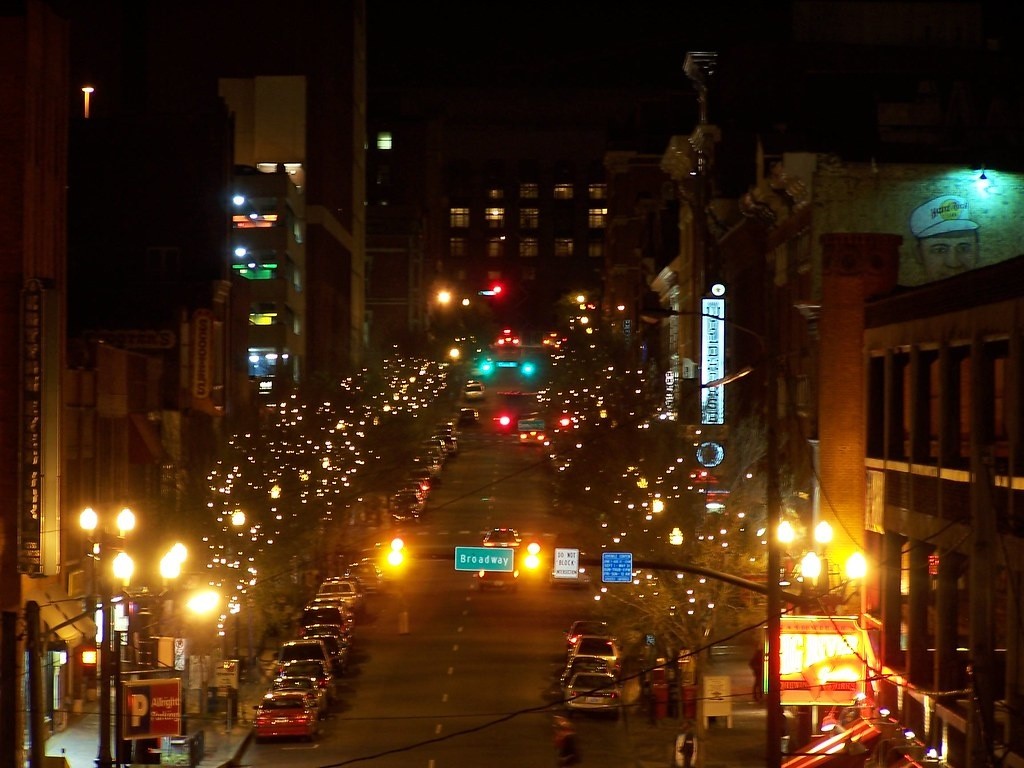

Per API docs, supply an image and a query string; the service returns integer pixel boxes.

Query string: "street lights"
[775,519,870,742]
[112,542,188,768]
[79,505,136,766]
[24,591,219,768]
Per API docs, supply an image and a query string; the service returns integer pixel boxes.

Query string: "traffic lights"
[560,417,569,425]
[500,415,510,426]
[384,536,406,568]
[524,539,543,571]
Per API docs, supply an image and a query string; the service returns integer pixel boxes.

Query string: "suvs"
[562,619,612,657]
[560,654,617,695]
[553,532,591,560]
[274,640,332,674]
[564,670,622,717]
[570,634,621,674]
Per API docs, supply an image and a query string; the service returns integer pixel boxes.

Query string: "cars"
[550,564,591,589]
[478,568,519,589]
[268,678,327,717]
[291,378,486,669]
[299,633,346,672]
[519,430,545,444]
[278,659,335,699]
[481,528,523,556]
[252,691,318,738]
